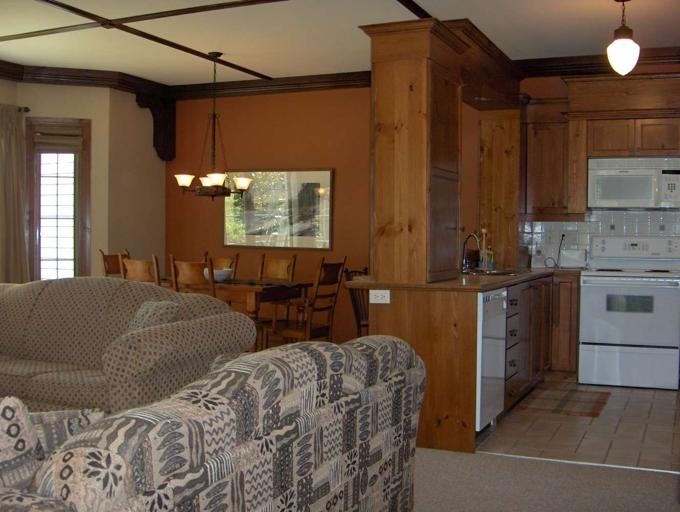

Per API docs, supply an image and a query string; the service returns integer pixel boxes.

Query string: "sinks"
[467,270,522,276]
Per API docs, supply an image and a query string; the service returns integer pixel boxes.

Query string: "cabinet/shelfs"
[587,73,680,157]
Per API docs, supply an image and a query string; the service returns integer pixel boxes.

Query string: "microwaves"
[586,156,680,209]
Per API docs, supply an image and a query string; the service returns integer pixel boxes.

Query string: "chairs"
[99,248,369,353]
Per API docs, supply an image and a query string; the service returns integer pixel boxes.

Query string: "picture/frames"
[222,168,335,251]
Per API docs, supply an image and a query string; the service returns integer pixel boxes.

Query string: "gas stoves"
[582,259,680,276]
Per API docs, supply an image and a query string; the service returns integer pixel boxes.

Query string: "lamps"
[607,0,640,76]
[174,51,253,201]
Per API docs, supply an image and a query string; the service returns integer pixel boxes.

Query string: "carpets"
[518,387,611,418]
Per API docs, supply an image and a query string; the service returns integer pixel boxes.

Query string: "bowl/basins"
[203,268,233,282]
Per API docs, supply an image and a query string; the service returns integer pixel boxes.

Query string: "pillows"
[0,395,105,492]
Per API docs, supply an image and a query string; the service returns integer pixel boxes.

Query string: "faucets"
[464,232,482,275]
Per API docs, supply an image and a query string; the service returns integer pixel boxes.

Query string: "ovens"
[579,275,679,348]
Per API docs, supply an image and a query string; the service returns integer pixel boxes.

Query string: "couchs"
[0,276,257,417]
[0,334,425,512]
